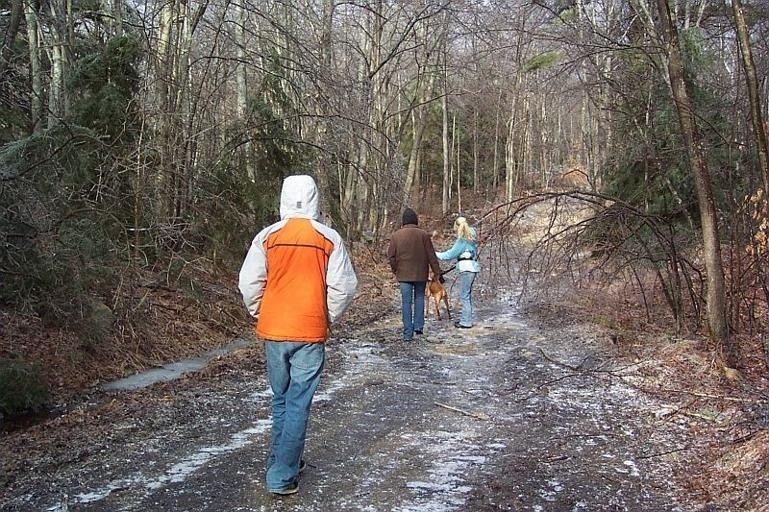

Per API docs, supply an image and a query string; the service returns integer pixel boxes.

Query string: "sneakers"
[404,329,423,340]
[268,460,306,494]
[454,321,471,329]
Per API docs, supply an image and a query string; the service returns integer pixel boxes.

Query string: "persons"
[435,217,481,327]
[387,207,441,341]
[238,174,358,494]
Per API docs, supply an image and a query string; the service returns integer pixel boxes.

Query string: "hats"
[402,208,419,226]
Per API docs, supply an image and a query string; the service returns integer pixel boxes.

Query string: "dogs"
[424,269,452,321]
